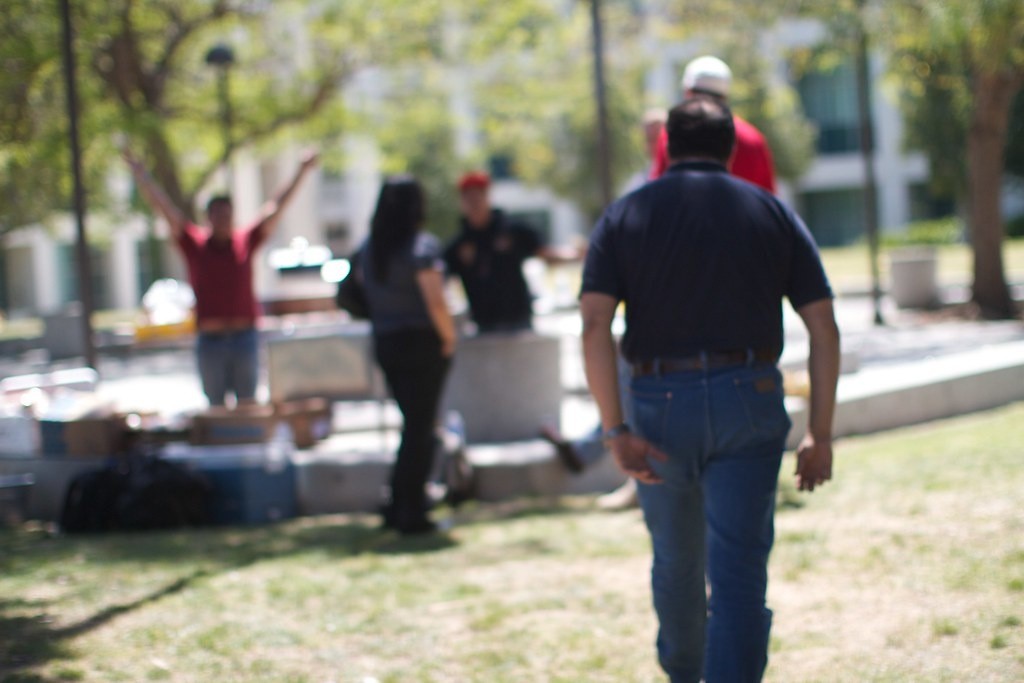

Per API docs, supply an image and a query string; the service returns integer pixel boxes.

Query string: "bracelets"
[599,424,629,441]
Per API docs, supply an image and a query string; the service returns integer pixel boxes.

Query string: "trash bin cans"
[890,245,940,311]
[41,306,87,363]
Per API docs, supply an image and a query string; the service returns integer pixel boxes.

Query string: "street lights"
[207,45,241,199]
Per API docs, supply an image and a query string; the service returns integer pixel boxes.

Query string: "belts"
[634,350,775,375]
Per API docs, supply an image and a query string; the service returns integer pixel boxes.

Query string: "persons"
[579,97,840,683]
[595,56,778,511]
[438,172,588,334]
[358,180,455,536]
[541,106,666,473]
[120,144,320,406]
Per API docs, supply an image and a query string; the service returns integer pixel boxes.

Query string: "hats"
[681,55,732,98]
[458,172,489,190]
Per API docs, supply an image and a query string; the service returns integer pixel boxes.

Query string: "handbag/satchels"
[336,251,371,313]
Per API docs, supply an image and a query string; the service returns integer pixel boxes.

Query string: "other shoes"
[540,424,583,472]
